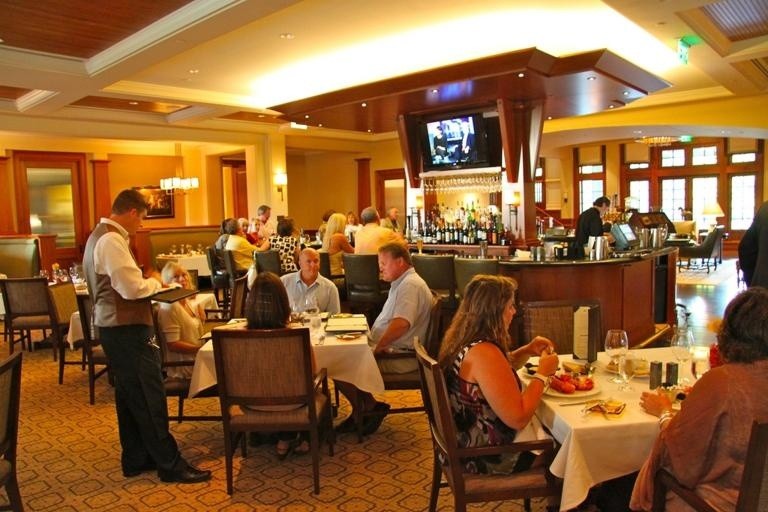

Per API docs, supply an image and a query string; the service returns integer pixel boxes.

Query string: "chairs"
[410,253,458,362]
[679,226,723,273]
[456,259,499,308]
[210,328,334,496]
[520,298,583,355]
[341,254,391,328]
[0,274,118,403]
[413,335,564,512]
[204,247,344,323]
[356,290,459,445]
[652,418,767,512]
[0,351,23,512]
[152,305,222,420]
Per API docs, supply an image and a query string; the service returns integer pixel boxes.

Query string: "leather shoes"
[277,449,288,459]
[161,466,211,482]
[334,403,389,434]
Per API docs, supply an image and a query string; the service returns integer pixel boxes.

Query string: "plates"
[603,367,651,378]
[519,367,538,378]
[653,384,693,411]
[543,378,602,398]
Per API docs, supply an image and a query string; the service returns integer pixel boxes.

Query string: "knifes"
[559,399,604,407]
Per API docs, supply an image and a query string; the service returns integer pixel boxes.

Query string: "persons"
[595,287,768,512]
[155,260,207,381]
[452,125,474,166]
[738,198,768,290]
[575,196,613,257]
[434,125,447,160]
[279,247,341,314]
[447,121,464,138]
[215,204,408,291]
[331,241,434,435]
[437,274,590,512]
[245,270,318,461]
[81,188,212,483]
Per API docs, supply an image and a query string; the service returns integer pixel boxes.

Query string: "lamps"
[636,135,676,148]
[415,196,424,231]
[703,201,724,232]
[159,177,200,196]
[275,171,287,192]
[514,191,521,230]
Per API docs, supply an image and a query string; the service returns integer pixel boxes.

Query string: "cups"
[346,231,349,241]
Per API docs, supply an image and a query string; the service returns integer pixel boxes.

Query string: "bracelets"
[658,409,674,430]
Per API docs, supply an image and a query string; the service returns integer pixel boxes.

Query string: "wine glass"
[670,330,695,384]
[39,267,78,283]
[304,296,320,316]
[605,329,629,384]
[309,317,322,343]
[417,239,423,256]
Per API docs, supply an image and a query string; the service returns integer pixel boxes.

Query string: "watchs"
[533,373,551,386]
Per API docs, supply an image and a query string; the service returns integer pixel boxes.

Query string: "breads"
[550,376,576,394]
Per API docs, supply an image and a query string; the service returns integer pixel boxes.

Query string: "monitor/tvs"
[610,223,640,250]
[417,111,491,172]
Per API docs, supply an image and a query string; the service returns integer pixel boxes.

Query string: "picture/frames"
[131,185,175,219]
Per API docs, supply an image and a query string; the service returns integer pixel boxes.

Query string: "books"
[324,316,370,332]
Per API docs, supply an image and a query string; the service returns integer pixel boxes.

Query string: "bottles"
[172,244,202,255]
[411,228,418,243]
[666,361,678,386]
[637,227,668,250]
[419,200,509,246]
[430,241,569,262]
[650,361,662,389]
[590,249,595,260]
[595,236,609,260]
